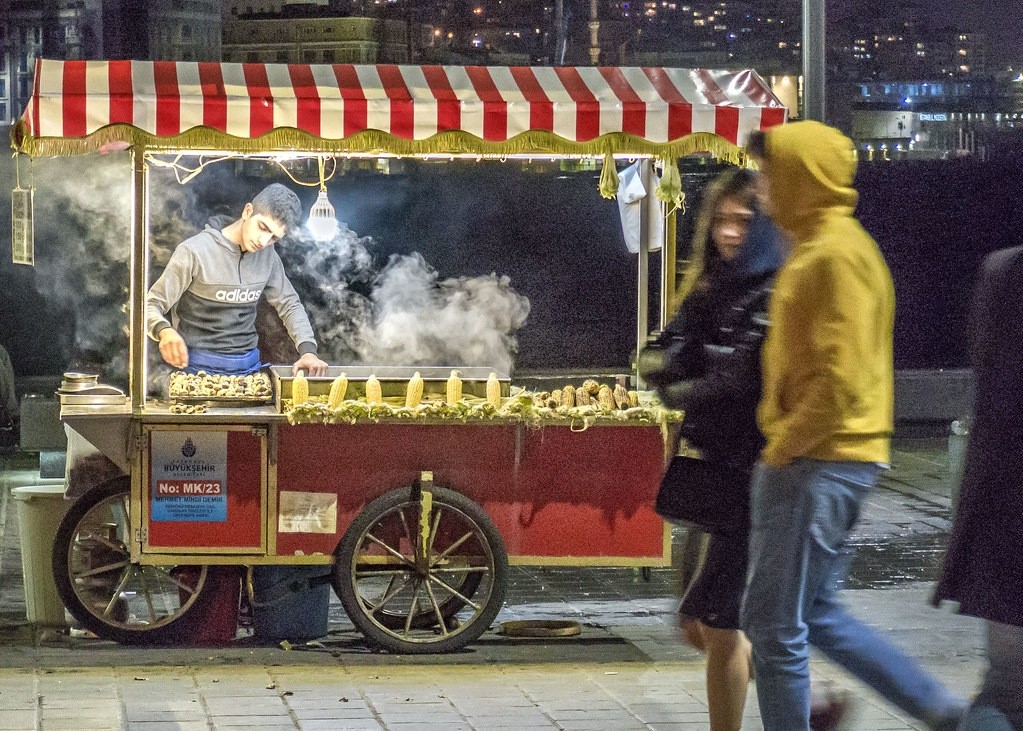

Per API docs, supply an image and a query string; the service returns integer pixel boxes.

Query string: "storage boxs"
[270,364,512,400]
[251,563,333,640]
[169,563,249,647]
[10,484,130,631]
[168,388,273,408]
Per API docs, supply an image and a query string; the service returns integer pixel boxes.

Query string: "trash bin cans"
[10,483,111,627]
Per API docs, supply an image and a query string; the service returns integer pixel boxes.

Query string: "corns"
[293,371,638,412]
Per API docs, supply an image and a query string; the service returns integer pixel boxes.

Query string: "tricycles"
[11,51,791,655]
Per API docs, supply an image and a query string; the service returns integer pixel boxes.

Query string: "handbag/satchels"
[657,454,753,538]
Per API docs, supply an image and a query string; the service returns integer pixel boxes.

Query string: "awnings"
[13,51,790,408]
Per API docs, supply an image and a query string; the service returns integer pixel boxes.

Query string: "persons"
[744,120,968,730]
[638,164,848,730]
[145,182,330,379]
[928,243,1023,729]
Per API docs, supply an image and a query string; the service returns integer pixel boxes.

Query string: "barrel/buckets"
[246,566,330,640]
[175,565,241,642]
[12,484,113,624]
